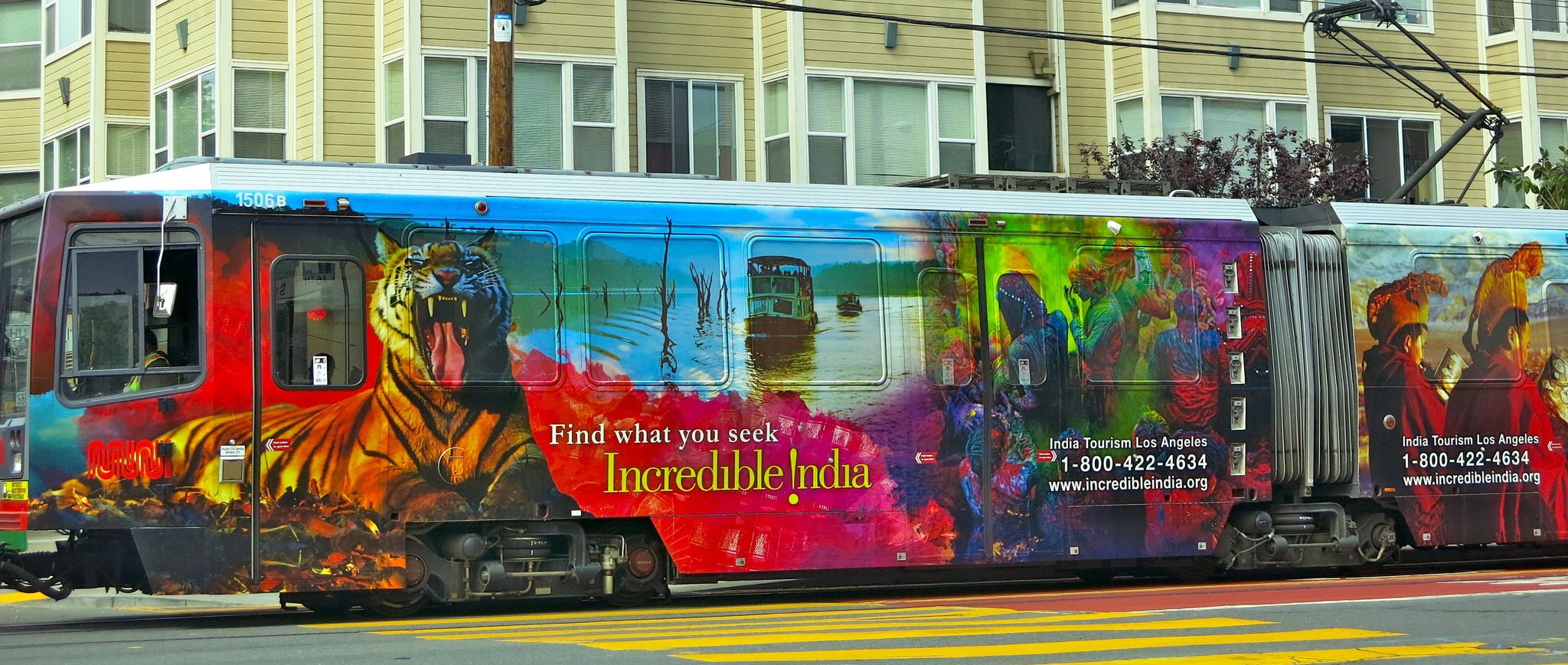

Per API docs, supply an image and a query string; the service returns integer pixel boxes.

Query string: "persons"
[120,328,172,395]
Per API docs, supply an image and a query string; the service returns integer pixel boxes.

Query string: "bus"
[0,0,1568,621]
